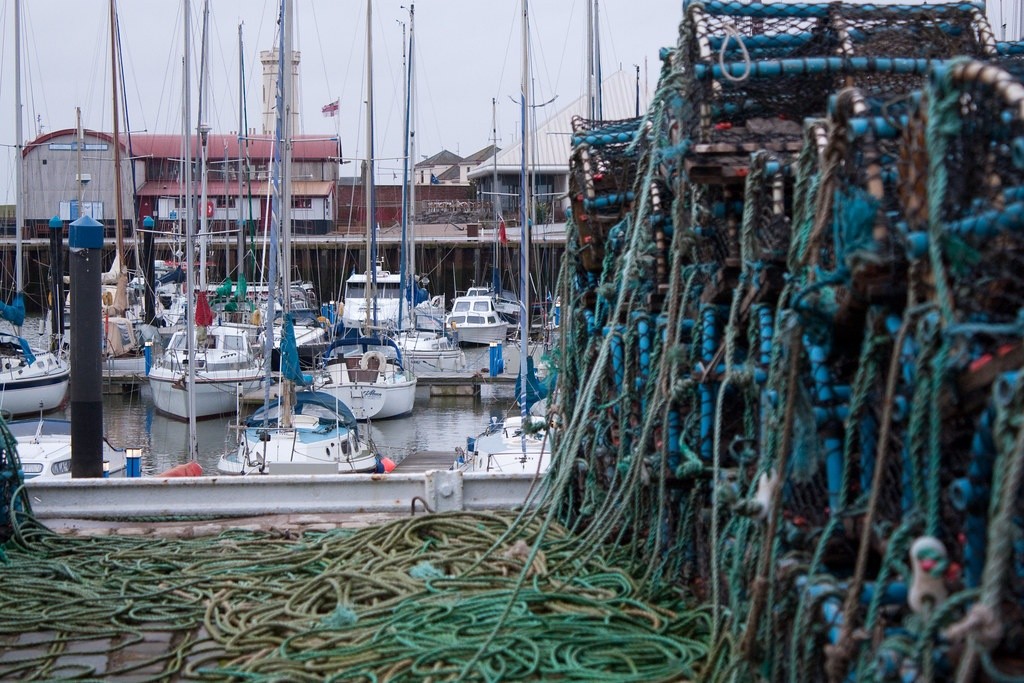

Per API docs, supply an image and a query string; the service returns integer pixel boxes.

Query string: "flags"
[322,98,340,117]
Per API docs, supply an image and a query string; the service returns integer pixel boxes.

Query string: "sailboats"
[0,1,647,477]
